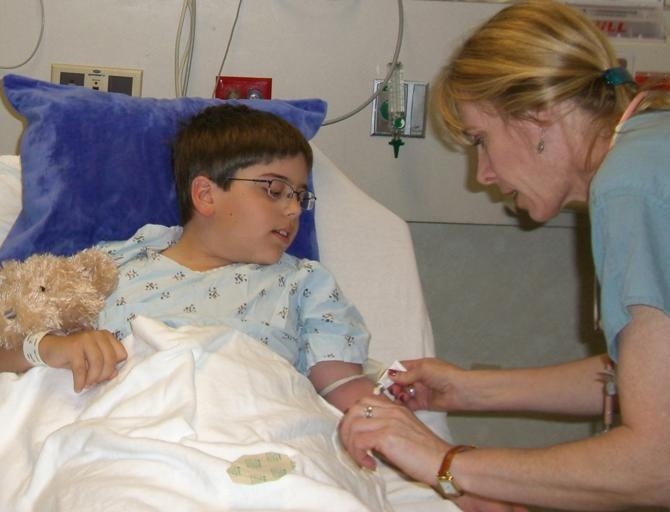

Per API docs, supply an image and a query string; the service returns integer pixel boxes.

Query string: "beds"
[0,148,465,511]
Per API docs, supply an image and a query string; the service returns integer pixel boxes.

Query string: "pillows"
[1,74,327,266]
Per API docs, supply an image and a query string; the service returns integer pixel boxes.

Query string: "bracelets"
[22,329,52,368]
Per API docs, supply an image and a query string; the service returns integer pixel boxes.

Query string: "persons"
[338,0,669,512]
[0,104,530,511]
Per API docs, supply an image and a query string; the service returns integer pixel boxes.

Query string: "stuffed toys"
[0,245,119,353]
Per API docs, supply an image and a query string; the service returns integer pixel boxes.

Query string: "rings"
[406,385,415,396]
[365,404,374,417]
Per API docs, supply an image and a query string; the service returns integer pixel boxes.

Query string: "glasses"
[209,176,317,212]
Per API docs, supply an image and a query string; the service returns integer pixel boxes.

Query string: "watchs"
[437,443,477,499]
